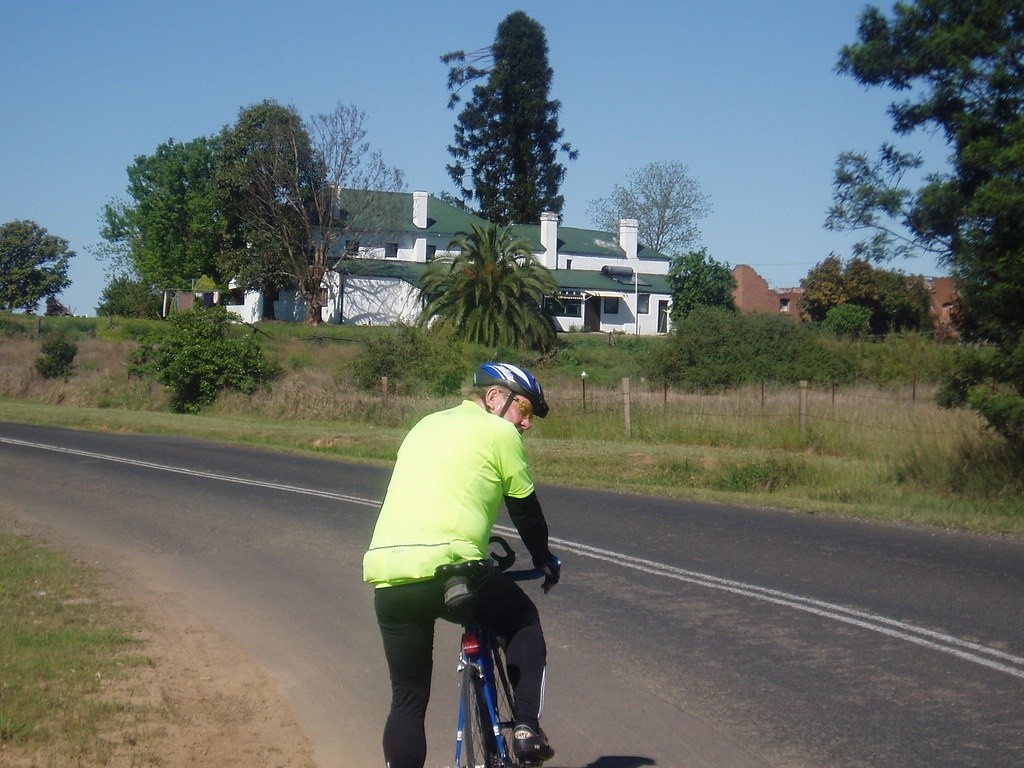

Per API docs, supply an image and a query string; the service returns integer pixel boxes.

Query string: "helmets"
[473,363,549,418]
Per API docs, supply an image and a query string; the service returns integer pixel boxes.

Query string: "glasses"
[499,390,533,422]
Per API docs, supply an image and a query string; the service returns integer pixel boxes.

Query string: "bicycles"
[452,534,546,768]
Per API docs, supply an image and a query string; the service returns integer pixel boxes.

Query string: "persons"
[362,362,561,768]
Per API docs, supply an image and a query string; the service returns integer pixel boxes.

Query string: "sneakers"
[513,721,555,760]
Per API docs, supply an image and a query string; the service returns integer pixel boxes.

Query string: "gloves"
[532,548,561,594]
